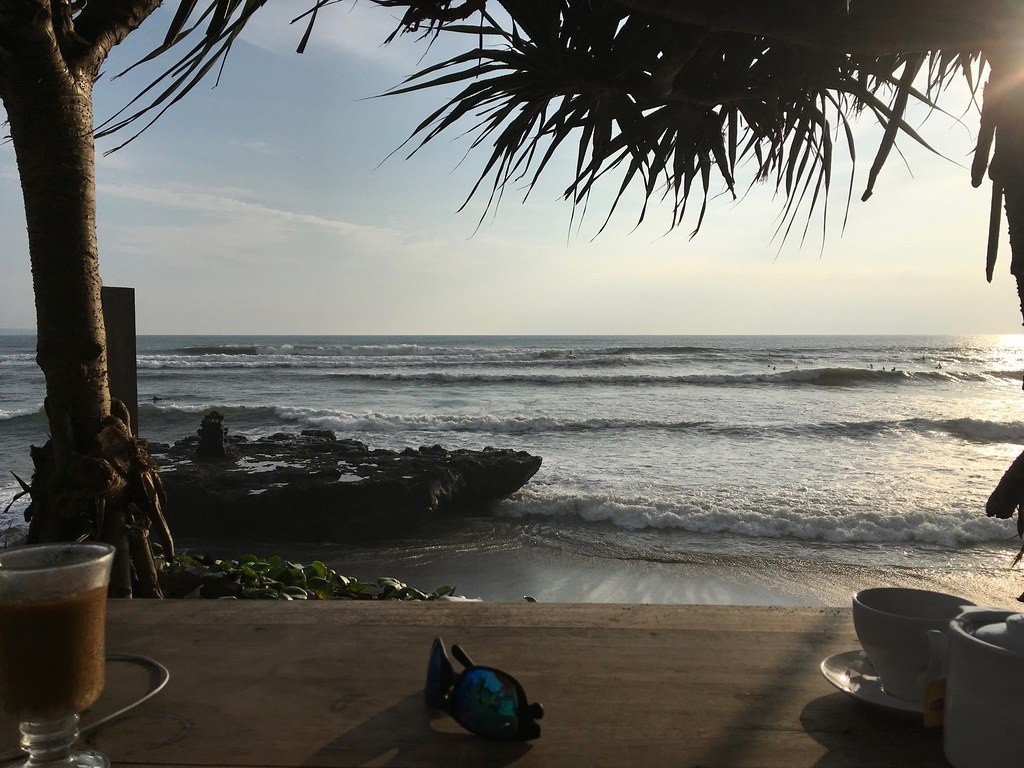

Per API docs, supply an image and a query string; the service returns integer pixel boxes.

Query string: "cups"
[852,588,977,702]
[942,605,1024,768]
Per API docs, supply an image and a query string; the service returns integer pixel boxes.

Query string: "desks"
[0,600,957,768]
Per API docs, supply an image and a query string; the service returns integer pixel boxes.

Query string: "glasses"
[424,636,545,743]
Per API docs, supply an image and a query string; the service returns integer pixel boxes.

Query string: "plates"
[818,646,926,716]
[0,650,169,761]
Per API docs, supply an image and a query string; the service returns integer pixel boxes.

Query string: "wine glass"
[0,542,117,768]
